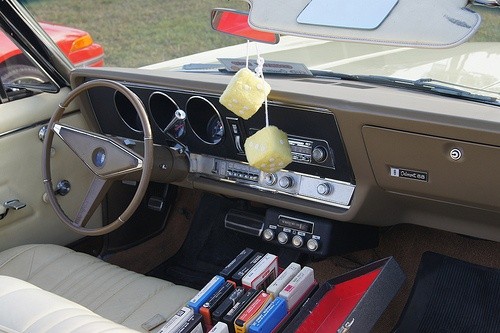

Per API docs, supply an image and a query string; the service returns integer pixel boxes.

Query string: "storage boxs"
[270,255,407,333]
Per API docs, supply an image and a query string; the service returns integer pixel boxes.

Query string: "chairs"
[0,244,200,333]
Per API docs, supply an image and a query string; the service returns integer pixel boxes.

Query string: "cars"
[0,19,105,103]
